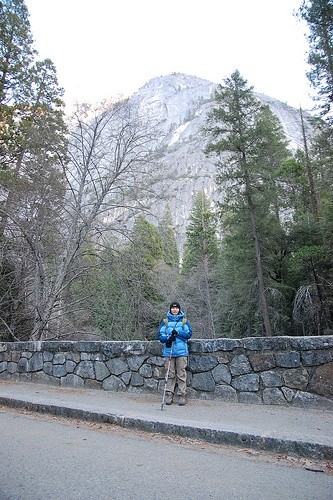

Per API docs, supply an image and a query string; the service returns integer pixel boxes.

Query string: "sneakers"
[165,397,173,405]
[179,397,185,405]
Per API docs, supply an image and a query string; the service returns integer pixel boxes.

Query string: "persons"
[158,301,192,406]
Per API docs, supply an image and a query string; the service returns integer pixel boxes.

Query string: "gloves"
[169,336,176,340]
[172,330,178,335]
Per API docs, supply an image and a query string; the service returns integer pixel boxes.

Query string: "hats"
[170,302,180,309]
